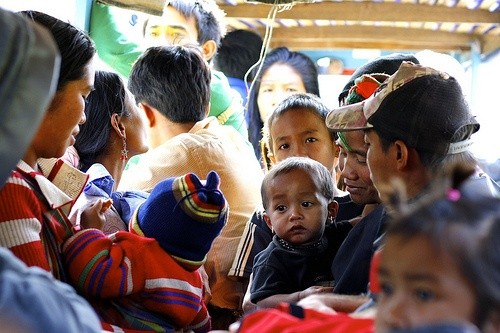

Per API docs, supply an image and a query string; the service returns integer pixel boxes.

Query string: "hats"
[129,171,230,272]
[336,52,419,103]
[326,61,481,171]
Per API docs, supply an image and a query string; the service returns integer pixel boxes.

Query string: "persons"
[1,1,500,333]
